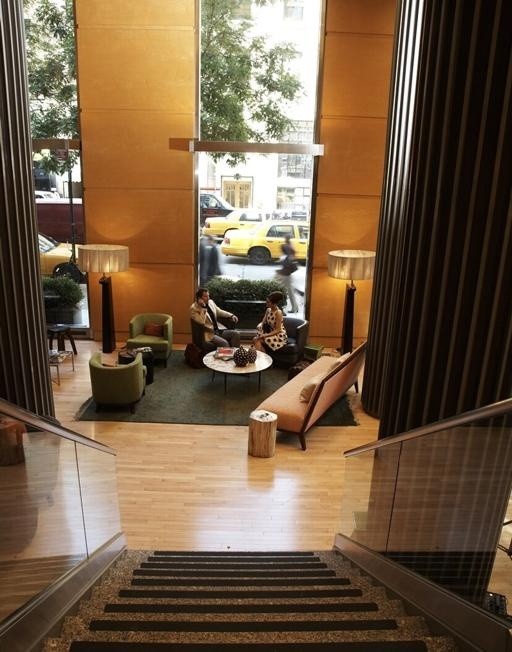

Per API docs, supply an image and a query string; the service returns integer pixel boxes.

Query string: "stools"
[47,326,78,356]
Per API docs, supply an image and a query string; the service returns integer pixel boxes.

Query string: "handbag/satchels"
[262,322,272,333]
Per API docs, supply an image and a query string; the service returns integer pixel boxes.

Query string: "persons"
[253,291,288,353]
[190,288,241,348]
[200,227,307,313]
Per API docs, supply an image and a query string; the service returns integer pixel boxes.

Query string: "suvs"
[199,194,234,226]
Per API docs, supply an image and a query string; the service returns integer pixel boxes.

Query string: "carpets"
[72,349,359,427]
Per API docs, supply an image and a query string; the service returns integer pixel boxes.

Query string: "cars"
[202,207,282,239]
[291,204,307,219]
[37,231,86,284]
[35,190,59,198]
[222,219,311,265]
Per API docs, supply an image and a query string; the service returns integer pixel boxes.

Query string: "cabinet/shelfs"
[303,342,324,362]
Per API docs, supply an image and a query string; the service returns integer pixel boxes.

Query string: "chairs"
[128,313,173,368]
[190,314,235,348]
[88,353,147,413]
[260,318,309,367]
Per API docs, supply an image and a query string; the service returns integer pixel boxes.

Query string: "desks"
[47,350,74,385]
[247,409,278,457]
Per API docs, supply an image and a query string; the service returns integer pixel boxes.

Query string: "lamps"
[328,248,378,394]
[78,243,129,353]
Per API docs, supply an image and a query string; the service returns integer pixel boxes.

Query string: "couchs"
[255,339,372,450]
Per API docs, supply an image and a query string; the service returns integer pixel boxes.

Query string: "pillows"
[299,372,327,402]
[143,317,165,337]
[328,352,350,373]
